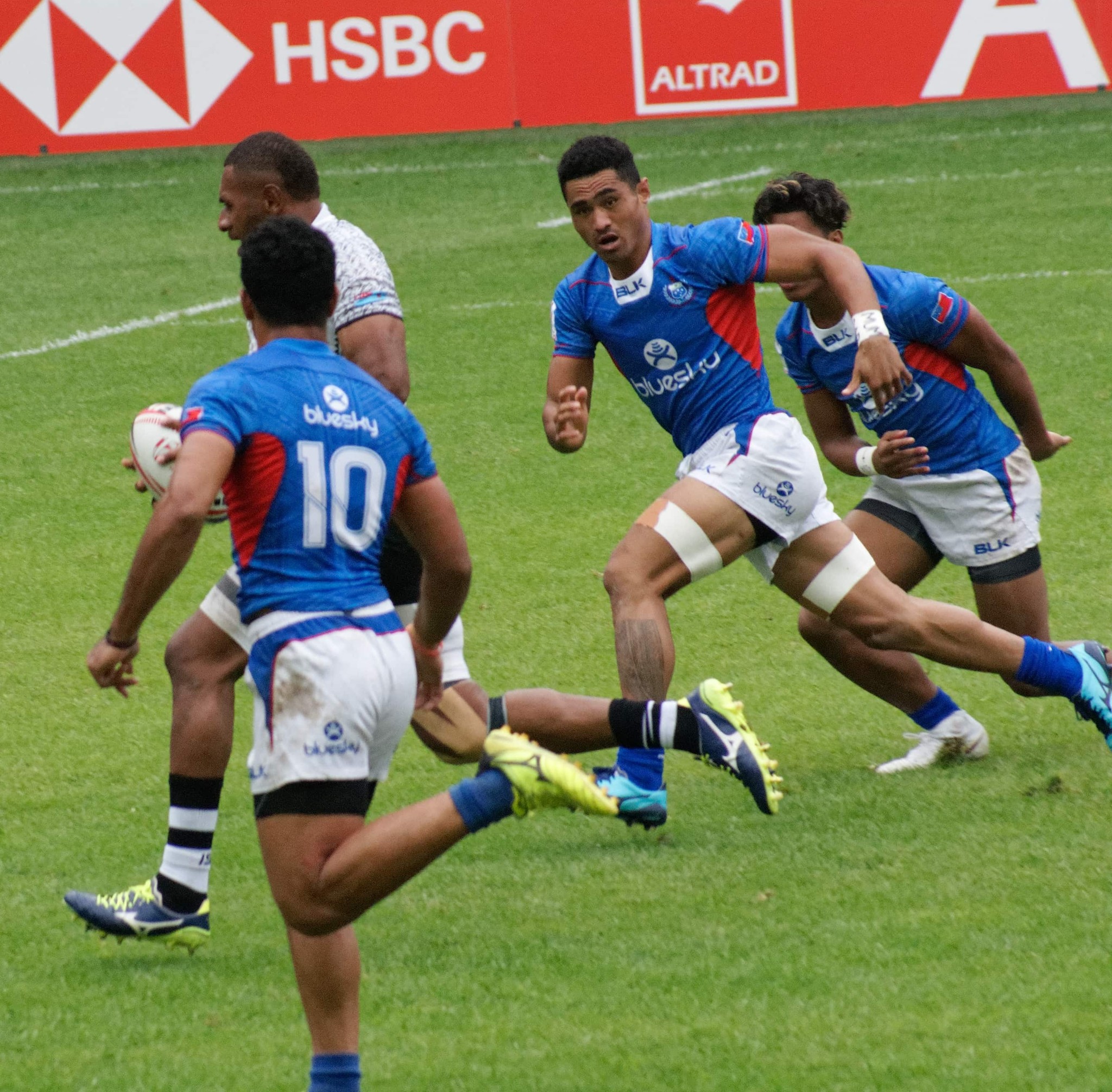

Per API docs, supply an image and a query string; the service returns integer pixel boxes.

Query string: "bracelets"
[406,624,443,656]
[856,446,882,475]
[107,629,138,648]
[852,310,891,346]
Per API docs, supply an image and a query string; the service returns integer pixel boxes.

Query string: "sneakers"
[873,711,990,775]
[474,723,619,823]
[685,678,782,815]
[1072,641,1112,753]
[591,765,666,829]
[62,878,212,957]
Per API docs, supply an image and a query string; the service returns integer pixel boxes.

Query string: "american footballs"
[128,402,231,523]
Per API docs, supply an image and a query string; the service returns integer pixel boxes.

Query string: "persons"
[65,132,781,955]
[86,214,617,1092]
[750,170,1112,775]
[542,135,1112,825]
